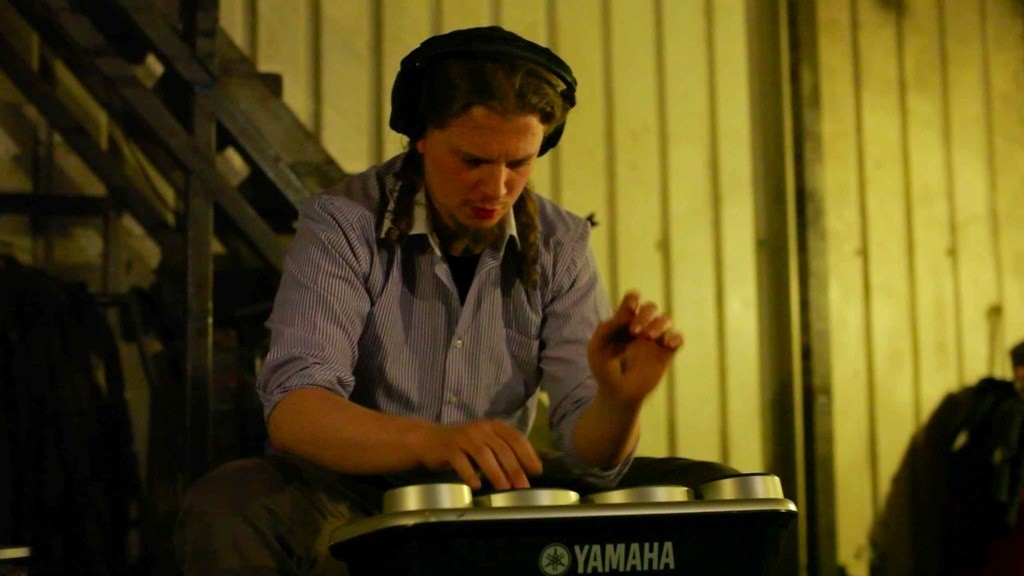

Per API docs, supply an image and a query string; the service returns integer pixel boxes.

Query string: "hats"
[389,26,578,158]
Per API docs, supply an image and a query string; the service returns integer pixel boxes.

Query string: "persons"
[173,25,743,576]
